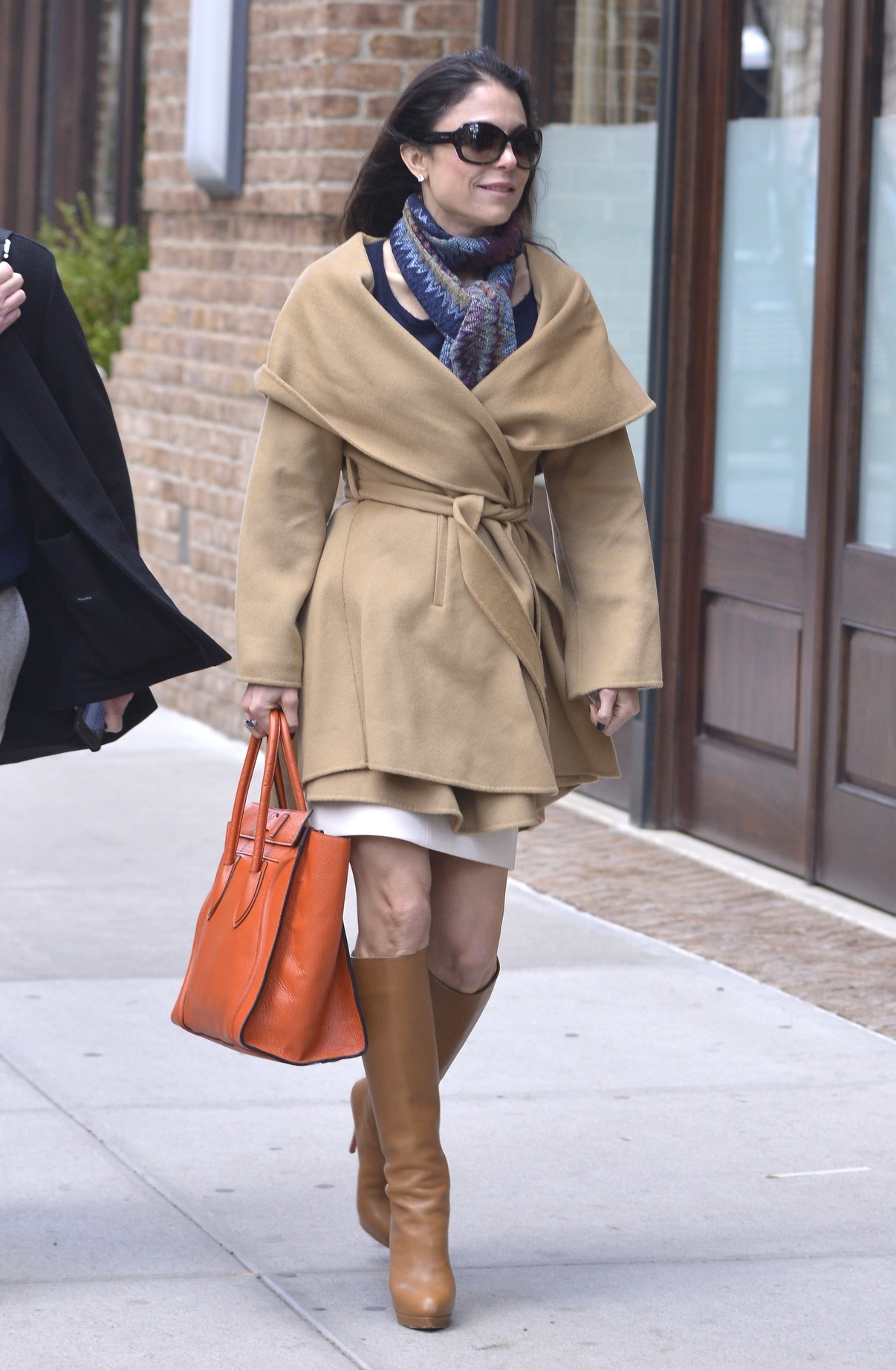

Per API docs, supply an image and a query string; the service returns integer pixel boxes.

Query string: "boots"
[350,955,500,1248]
[350,944,456,1329]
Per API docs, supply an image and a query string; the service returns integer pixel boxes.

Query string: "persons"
[0,227,232,767]
[235,45,665,1331]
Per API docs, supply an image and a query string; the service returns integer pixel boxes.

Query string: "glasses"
[419,121,543,170]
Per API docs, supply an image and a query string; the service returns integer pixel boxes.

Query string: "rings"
[243,719,258,727]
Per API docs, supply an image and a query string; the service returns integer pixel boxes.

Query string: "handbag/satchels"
[170,707,368,1066]
